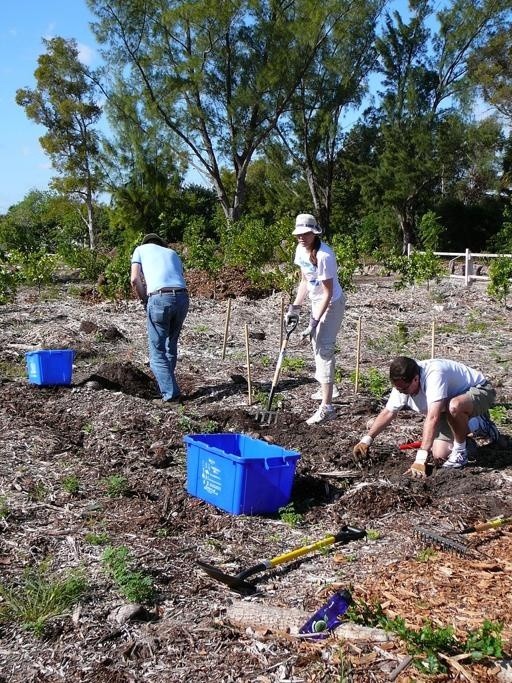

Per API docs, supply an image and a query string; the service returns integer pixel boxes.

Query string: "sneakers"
[151,399,179,408]
[305,405,337,426]
[442,449,468,467]
[311,385,340,399]
[476,410,500,441]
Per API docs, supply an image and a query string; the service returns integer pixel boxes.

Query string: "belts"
[149,288,188,297]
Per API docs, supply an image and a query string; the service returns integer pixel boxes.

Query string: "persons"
[285,214,344,425]
[356,356,496,478]
[131,232,189,401]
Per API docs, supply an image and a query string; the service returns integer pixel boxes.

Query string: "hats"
[143,233,163,244]
[291,214,322,236]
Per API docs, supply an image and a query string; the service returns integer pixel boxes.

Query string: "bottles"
[299,589,353,641]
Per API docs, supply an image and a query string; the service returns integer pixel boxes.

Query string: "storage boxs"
[182,432,301,516]
[24,349,75,386]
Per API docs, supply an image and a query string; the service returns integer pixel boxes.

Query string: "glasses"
[396,383,411,391]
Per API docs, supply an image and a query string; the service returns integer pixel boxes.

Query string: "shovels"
[194,523,367,595]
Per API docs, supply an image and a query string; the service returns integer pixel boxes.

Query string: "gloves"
[353,436,373,462]
[403,450,428,478]
[301,319,319,342]
[286,304,302,327]
